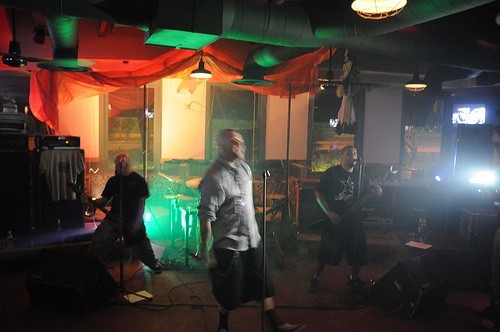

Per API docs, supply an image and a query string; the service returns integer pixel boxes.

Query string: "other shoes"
[278,323,308,332]
[152,261,162,273]
[347,275,361,288]
[309,277,320,292]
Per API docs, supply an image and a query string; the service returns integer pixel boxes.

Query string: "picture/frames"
[399,90,452,183]
[205,81,266,177]
[300,81,365,179]
[99,80,163,173]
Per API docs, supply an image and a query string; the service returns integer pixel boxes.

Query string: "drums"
[260,207,283,243]
[179,202,197,236]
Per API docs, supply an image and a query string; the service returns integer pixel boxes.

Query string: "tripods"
[96,170,153,310]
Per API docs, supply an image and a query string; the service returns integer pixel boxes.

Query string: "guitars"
[327,183,382,228]
[66,183,146,248]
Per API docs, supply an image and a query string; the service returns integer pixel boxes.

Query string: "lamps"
[402,62,428,93]
[191,50,212,80]
[350,0,408,22]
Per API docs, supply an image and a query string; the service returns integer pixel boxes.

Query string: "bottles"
[7,230,14,248]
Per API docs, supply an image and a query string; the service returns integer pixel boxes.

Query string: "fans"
[0,6,57,68]
[319,49,390,94]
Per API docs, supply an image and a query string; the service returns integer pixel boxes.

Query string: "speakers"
[365,260,449,319]
[0,149,34,235]
[32,149,84,230]
[25,251,118,315]
[290,186,329,242]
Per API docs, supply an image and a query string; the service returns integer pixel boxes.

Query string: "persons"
[308,145,382,293]
[79,154,163,274]
[196,129,305,331]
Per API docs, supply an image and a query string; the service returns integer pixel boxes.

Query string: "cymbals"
[187,177,203,188]
[265,193,286,199]
[164,194,194,199]
